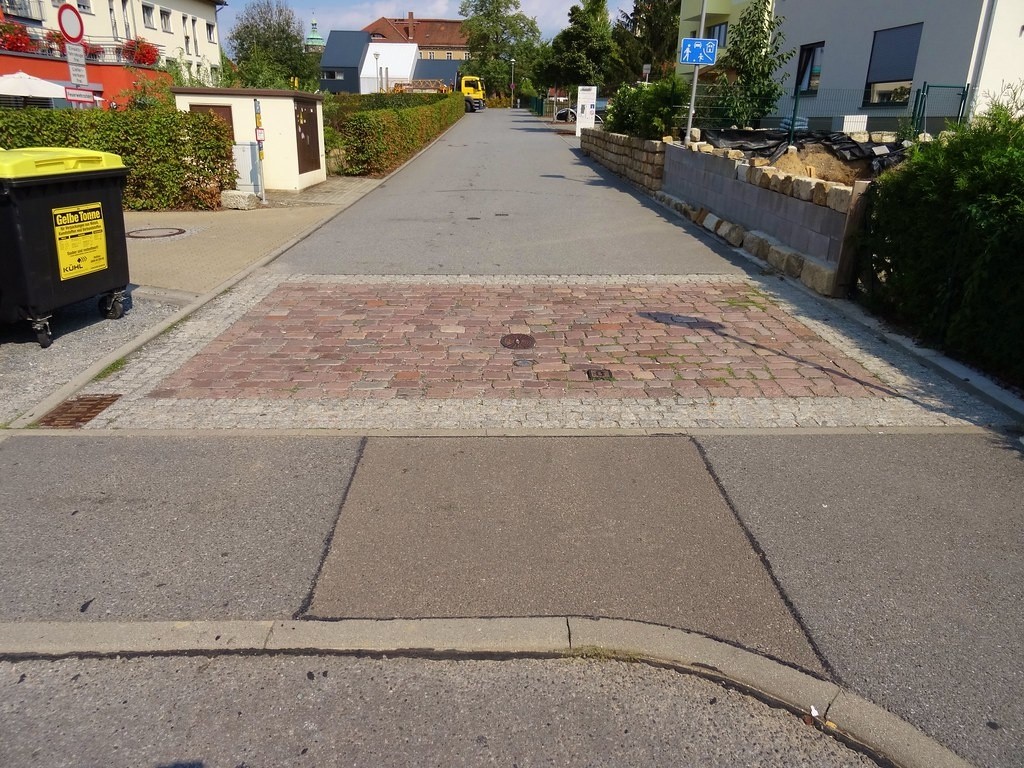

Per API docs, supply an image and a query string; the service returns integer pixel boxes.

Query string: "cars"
[555,97,610,122]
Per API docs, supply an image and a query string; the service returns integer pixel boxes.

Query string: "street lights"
[510,59,516,107]
[373,51,381,94]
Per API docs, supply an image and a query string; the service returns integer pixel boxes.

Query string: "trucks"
[389,72,487,112]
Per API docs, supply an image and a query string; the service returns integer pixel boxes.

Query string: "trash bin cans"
[0,146,133,350]
